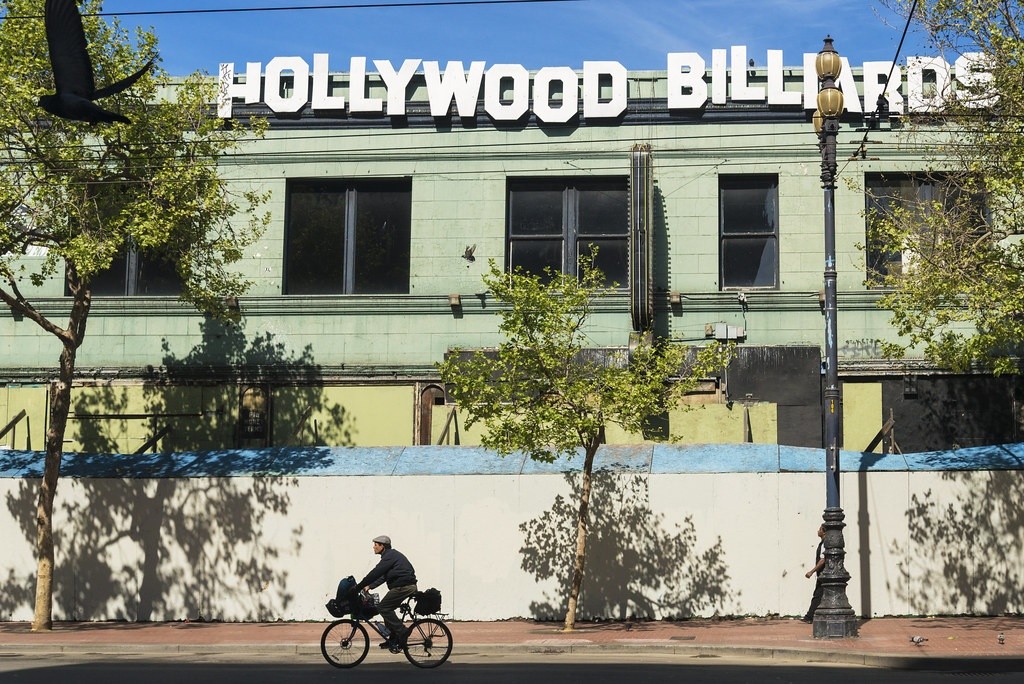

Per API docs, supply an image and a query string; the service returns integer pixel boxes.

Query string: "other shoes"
[398,627,411,645]
[380,640,394,647]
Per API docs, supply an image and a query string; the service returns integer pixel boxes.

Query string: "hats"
[373,536,391,544]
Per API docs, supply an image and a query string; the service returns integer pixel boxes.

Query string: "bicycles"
[320,589,454,670]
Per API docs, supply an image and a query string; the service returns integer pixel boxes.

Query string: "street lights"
[807,33,860,643]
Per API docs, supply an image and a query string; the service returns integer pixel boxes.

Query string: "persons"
[343,536,416,649]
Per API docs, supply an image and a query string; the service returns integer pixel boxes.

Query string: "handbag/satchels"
[415,587,441,616]
[359,591,379,619]
